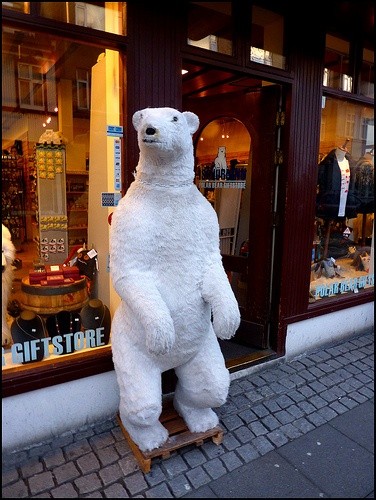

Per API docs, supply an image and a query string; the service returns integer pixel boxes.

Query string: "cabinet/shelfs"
[65,171,89,247]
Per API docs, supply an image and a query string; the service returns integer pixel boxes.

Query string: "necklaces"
[18,316,47,340]
[55,311,73,342]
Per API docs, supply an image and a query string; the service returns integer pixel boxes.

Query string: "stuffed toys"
[2,224,15,348]
[108,106,242,452]
